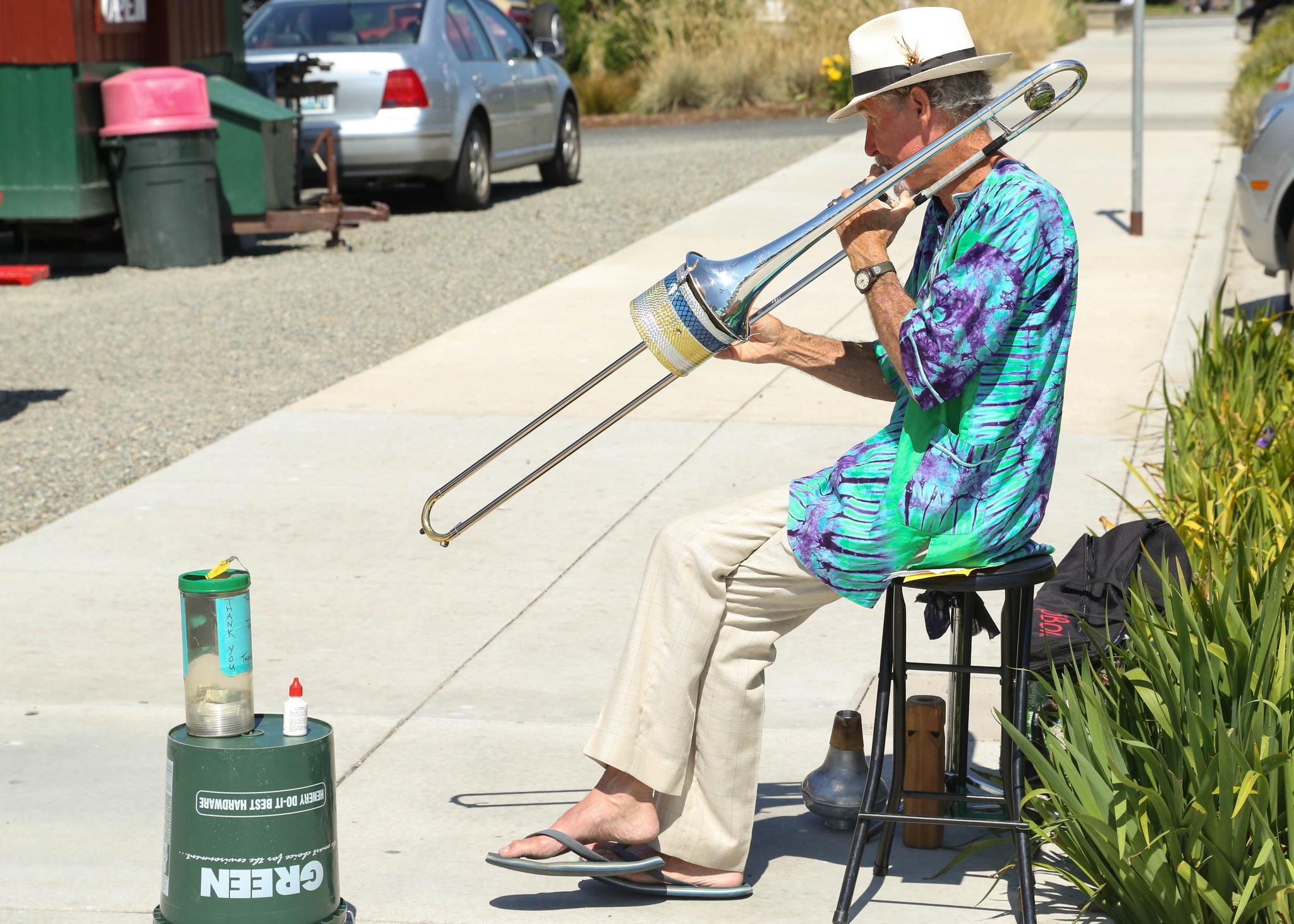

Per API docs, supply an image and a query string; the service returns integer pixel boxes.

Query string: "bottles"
[283,678,308,737]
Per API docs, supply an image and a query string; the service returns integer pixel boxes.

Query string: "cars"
[240,0,583,211]
[1235,63,1294,274]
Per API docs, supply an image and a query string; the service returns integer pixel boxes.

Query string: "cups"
[179,568,255,737]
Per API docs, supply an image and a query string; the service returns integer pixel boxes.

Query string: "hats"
[827,7,1011,123]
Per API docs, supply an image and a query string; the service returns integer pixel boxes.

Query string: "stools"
[830,549,1062,924]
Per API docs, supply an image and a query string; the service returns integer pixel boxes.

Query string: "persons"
[485,6,1079,897]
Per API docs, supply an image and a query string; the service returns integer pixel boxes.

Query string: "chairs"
[271,28,310,50]
[322,26,363,45]
[381,26,417,47]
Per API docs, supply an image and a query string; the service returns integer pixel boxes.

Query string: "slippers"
[486,830,665,876]
[593,873,752,899]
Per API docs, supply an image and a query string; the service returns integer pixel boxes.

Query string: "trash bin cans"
[94,66,222,270]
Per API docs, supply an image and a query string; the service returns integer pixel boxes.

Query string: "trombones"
[418,60,1089,546]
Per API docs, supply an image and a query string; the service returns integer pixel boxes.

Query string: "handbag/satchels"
[1024,520,1192,682]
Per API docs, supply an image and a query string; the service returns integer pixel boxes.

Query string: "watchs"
[855,261,896,294]
